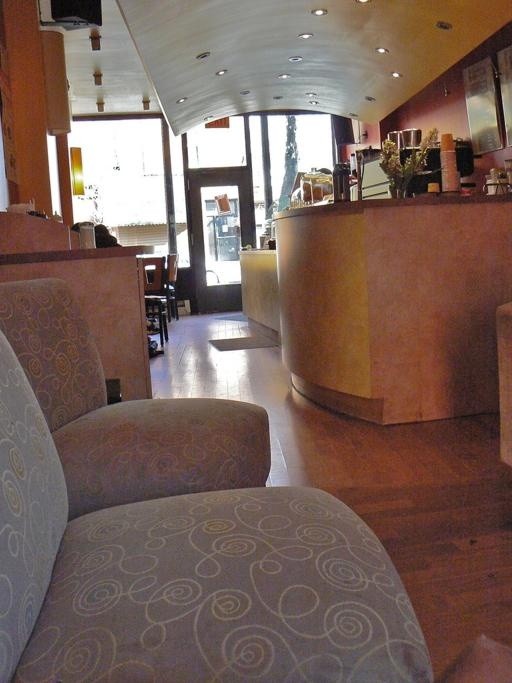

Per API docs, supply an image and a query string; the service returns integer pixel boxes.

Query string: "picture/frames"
[462,44,512,156]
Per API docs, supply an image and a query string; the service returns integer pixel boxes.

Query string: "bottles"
[350,151,356,175]
[79,221,96,249]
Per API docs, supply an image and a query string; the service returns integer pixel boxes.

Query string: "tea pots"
[300,168,333,201]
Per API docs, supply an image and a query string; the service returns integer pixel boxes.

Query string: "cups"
[427,183,439,193]
[440,133,459,192]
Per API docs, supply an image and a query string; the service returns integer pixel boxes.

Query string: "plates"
[486,181,508,194]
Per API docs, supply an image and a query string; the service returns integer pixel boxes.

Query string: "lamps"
[67,76,85,196]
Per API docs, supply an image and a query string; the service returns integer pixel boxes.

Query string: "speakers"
[50,0,102,31]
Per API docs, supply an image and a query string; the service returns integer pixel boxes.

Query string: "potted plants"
[378,126,439,199]
